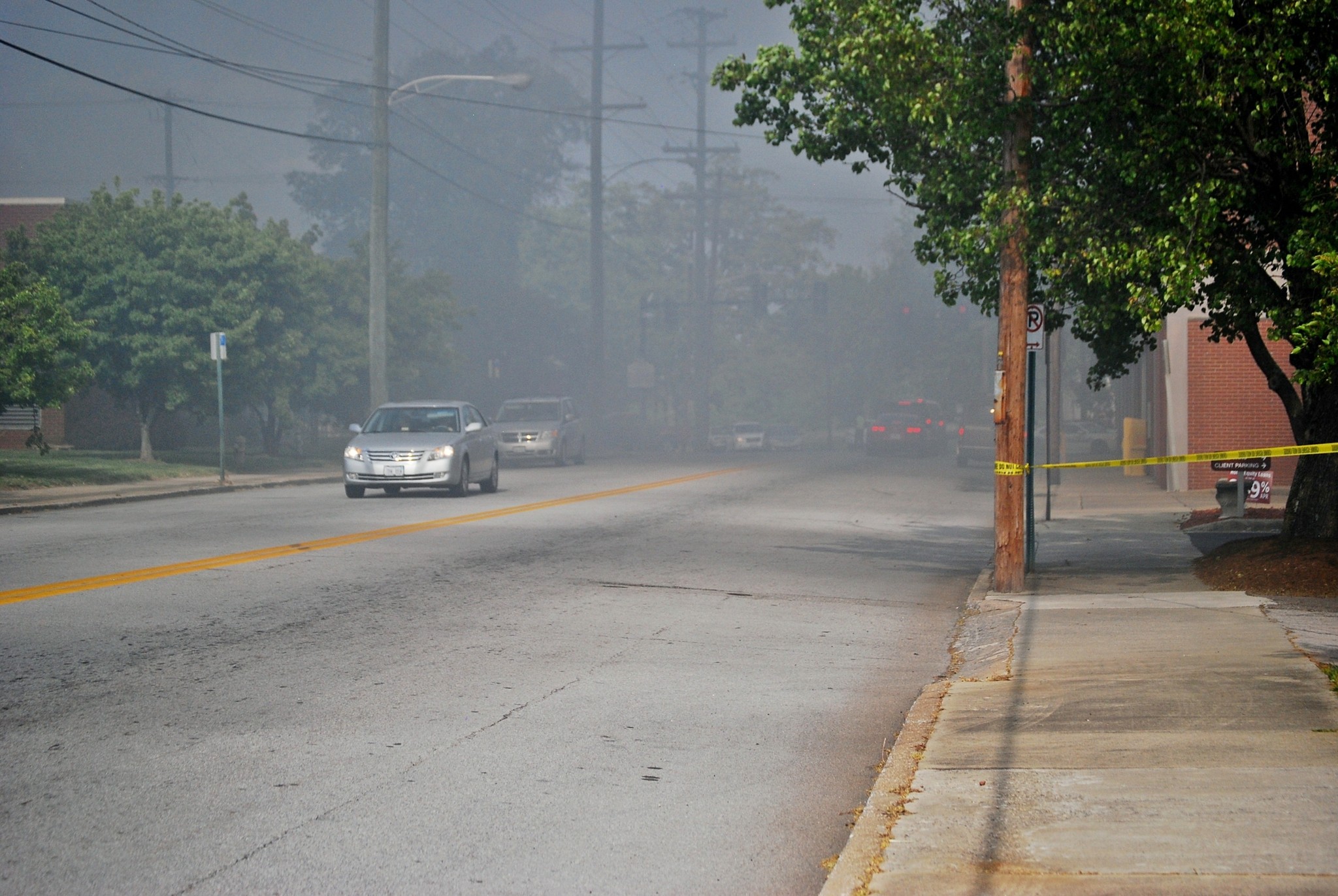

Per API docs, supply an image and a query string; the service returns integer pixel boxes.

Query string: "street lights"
[369,70,534,414]
[587,157,704,404]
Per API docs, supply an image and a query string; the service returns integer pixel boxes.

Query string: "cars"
[488,398,586,466]
[343,400,500,497]
[1033,420,1116,457]
[708,395,996,470]
